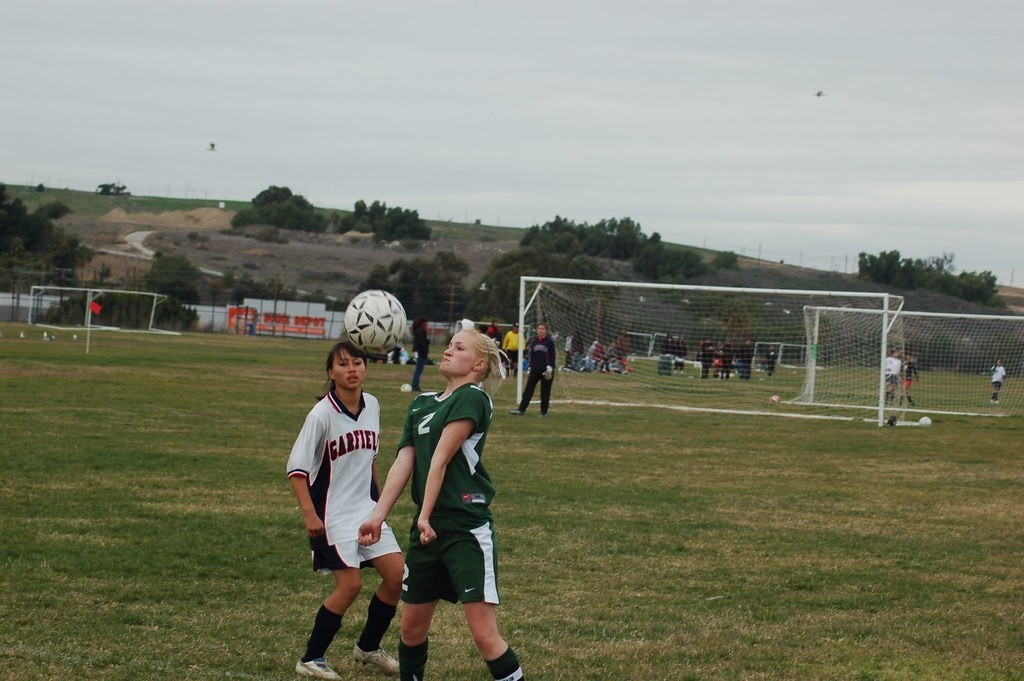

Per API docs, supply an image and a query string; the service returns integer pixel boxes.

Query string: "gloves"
[542,366,552,379]
[526,366,530,375]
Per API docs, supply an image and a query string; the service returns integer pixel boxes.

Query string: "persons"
[884,350,920,406]
[356,327,524,680]
[509,322,555,418]
[989,359,1006,405]
[287,340,406,680]
[388,315,635,393]
[657,332,779,380]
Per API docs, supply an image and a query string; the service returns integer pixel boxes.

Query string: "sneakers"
[295,658,341,680]
[353,642,400,676]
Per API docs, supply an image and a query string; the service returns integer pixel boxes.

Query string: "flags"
[90,301,102,315]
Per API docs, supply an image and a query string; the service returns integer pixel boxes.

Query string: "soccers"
[401,383,412,393]
[768,395,779,403]
[919,416,932,427]
[343,289,408,358]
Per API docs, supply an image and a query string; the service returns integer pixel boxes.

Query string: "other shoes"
[995,400,999,404]
[509,409,525,416]
[989,398,994,404]
[539,414,547,418]
[411,385,422,392]
